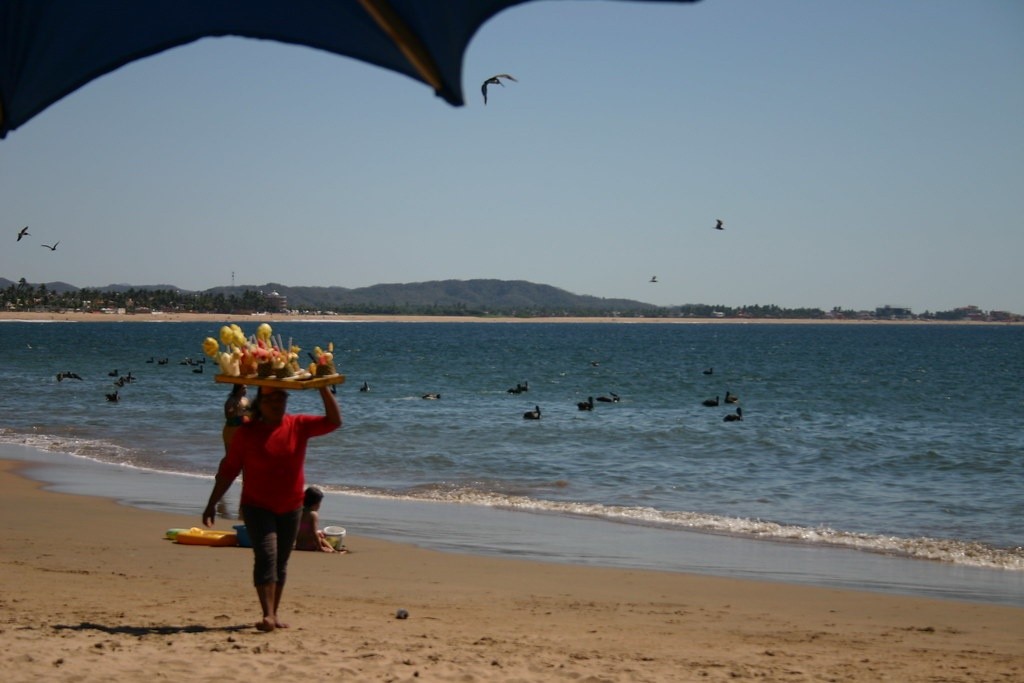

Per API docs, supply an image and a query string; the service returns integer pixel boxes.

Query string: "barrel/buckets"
[324,526,346,550]
[232,525,251,547]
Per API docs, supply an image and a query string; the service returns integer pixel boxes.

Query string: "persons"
[213,383,250,480]
[202,384,344,632]
[294,486,339,554]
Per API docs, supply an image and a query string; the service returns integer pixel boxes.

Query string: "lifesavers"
[177,527,238,546]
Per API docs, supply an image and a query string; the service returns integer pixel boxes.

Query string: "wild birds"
[41,240,60,251]
[17,226,32,241]
[354,217,745,426]
[57,355,206,401]
[480,74,518,105]
